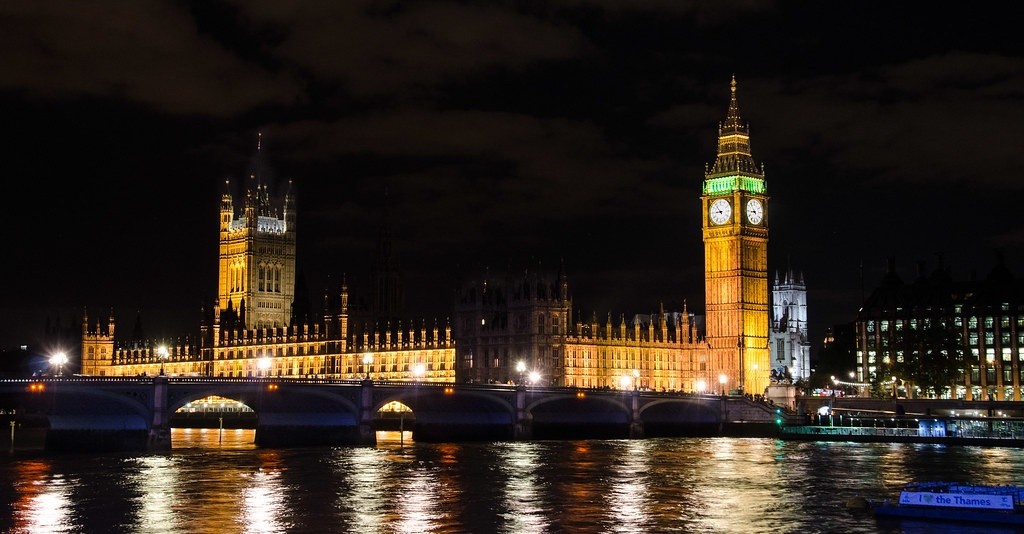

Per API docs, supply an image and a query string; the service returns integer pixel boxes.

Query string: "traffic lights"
[775,417,783,425]
[775,409,781,414]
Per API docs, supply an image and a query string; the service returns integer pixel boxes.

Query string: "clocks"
[746,198,764,225]
[708,198,731,225]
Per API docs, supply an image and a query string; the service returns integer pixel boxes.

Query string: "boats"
[873,480,1024,523]
